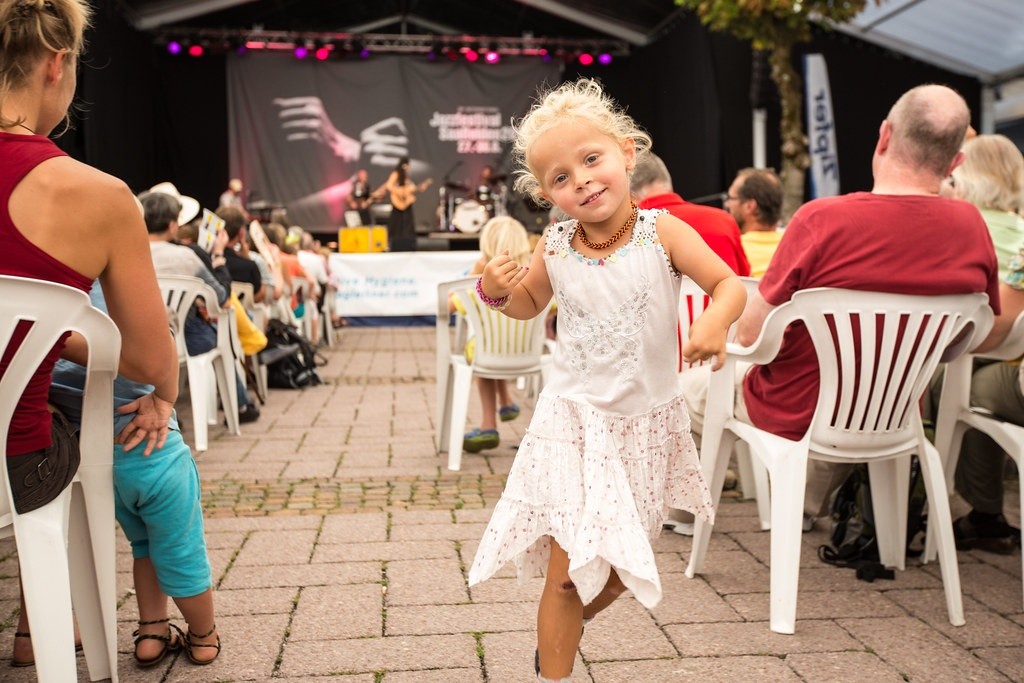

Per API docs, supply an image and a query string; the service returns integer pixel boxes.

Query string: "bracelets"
[475,277,512,311]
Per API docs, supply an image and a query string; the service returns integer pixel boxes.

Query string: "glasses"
[721,193,738,201]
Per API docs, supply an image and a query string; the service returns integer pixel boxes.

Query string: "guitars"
[390,178,432,211]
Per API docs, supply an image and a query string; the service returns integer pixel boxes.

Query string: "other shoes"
[224,402,260,426]
[169,416,183,432]
[803,516,817,531]
[663,508,695,535]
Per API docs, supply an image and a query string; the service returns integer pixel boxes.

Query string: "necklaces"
[575,199,638,249]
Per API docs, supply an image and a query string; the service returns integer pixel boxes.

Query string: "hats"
[150,182,200,227]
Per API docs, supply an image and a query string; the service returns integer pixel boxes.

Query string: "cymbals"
[484,174,508,183]
[444,181,471,192]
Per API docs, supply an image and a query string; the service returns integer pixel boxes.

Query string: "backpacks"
[816,419,935,583]
[266,319,328,389]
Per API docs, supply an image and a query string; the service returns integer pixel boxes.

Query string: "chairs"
[1,273,124,682]
[155,274,310,450]
[436,273,1024,636]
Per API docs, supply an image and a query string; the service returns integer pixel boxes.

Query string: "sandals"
[184,625,221,665]
[463,428,499,453]
[500,404,520,421]
[132,619,180,666]
[12,632,82,667]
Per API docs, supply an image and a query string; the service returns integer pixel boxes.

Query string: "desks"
[328,252,482,324]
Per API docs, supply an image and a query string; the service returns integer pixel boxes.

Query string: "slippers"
[953,512,1014,554]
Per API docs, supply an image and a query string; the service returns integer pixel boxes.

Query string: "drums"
[454,199,489,233]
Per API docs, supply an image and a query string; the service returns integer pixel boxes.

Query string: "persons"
[0,1,341,667]
[387,157,428,251]
[461,70,1024,682]
[346,169,386,226]
